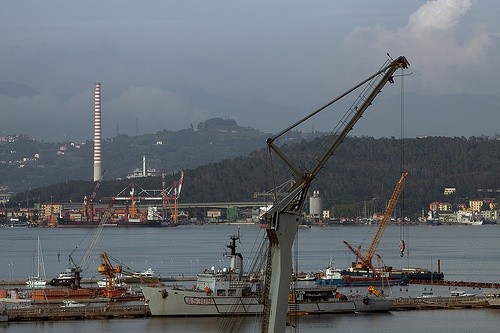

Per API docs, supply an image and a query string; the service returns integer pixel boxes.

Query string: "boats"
[140,283,395,318]
[0,213,500,317]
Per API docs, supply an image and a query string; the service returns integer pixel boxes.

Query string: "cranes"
[84,166,184,217]
[220,52,413,333]
[344,170,411,271]
